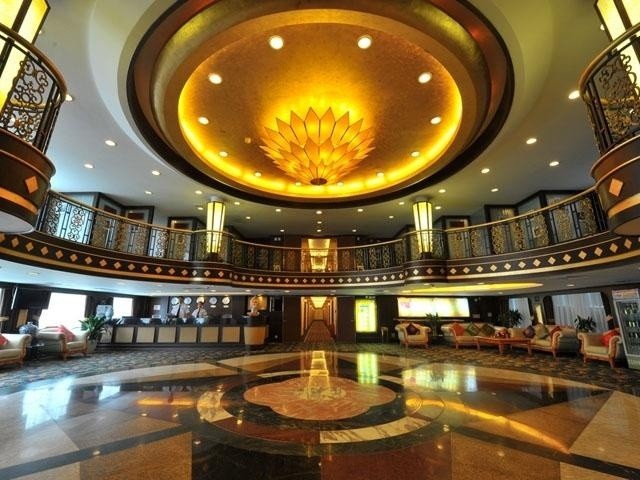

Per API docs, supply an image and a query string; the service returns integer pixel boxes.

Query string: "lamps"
[204,196,227,261]
[594,0,640,100]
[0,0,51,121]
[259,100,376,185]
[410,195,434,257]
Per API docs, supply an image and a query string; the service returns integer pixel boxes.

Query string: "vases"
[19,322,38,340]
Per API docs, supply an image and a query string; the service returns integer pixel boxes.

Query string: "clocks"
[170,296,231,305]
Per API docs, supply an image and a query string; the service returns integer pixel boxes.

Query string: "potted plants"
[425,312,441,345]
[79,314,108,352]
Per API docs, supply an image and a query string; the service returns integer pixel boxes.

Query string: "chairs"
[0,328,88,368]
[394,323,623,368]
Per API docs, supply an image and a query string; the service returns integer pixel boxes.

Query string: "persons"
[171,296,193,319]
[190,301,208,319]
[239,295,268,322]
